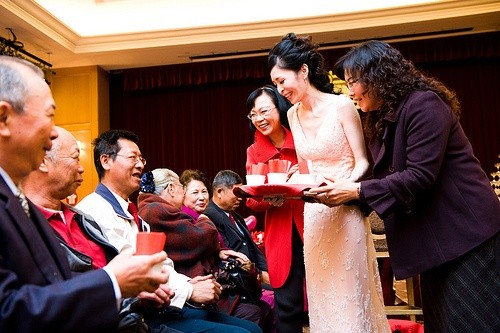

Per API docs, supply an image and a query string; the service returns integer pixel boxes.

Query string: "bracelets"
[357,183,362,196]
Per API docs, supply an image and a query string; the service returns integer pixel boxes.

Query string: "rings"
[324,193,329,199]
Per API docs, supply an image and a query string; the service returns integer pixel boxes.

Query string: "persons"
[303,39,500,333]
[18,127,277,333]
[0,55,170,333]
[264,32,393,333]
[241,87,309,333]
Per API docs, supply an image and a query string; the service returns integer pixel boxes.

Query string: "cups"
[267,173,288,184]
[136,232,166,255]
[246,174,266,186]
[297,174,317,185]
[134,255,162,274]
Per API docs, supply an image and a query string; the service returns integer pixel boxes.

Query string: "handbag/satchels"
[223,259,262,302]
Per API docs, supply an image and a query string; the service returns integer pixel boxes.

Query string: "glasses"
[113,154,147,166]
[247,107,277,120]
[345,77,361,91]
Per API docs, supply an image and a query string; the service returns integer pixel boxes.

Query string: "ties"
[16,190,31,217]
[129,202,140,231]
[229,213,236,228]
